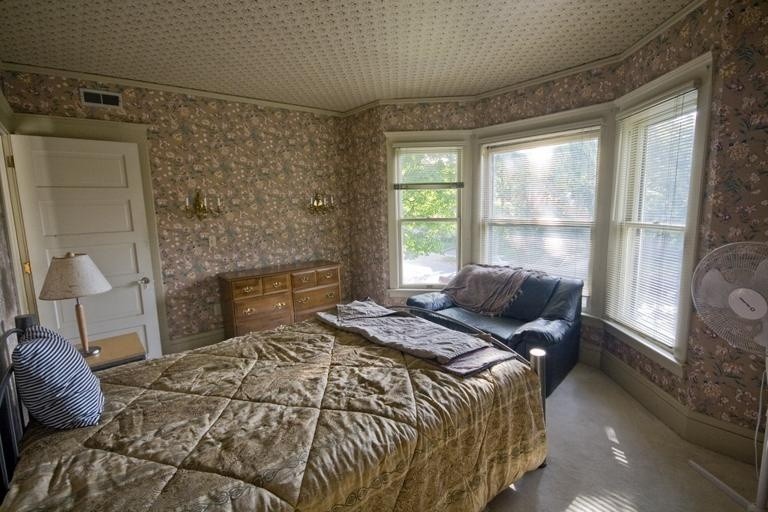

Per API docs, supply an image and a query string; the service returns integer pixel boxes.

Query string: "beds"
[1,298,547,511]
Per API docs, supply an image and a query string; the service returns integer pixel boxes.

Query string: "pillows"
[11,325,105,429]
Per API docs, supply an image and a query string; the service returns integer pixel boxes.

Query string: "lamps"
[184,190,225,221]
[307,193,338,216]
[38,252,113,356]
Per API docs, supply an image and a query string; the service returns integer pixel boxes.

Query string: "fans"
[687,240,768,512]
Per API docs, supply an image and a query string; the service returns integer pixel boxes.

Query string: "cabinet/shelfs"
[217,260,344,340]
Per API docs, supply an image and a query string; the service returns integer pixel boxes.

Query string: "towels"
[316,299,493,365]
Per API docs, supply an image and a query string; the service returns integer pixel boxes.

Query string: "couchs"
[407,262,584,398]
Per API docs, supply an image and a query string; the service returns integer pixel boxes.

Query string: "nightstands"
[76,331,147,372]
